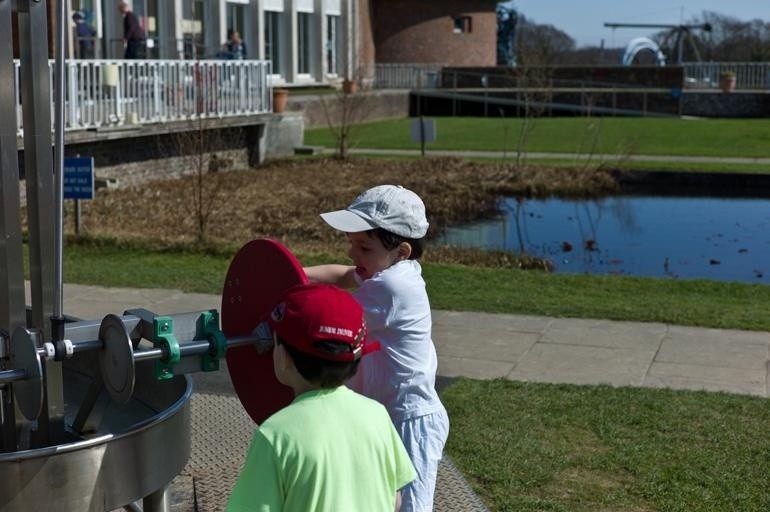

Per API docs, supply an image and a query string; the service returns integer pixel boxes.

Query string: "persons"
[222,285,418,510]
[73,13,96,58]
[301,185,450,511]
[118,0,148,59]
[223,29,247,61]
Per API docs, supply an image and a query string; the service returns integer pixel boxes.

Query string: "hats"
[269,284,383,361]
[319,184,430,240]
